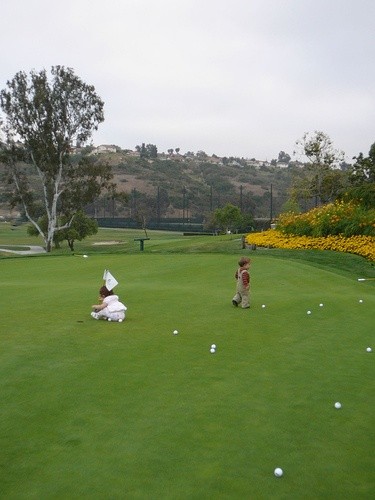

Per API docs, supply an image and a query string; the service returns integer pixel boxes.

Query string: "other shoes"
[90,312,100,320]
[241,306,250,309]
[232,300,238,308]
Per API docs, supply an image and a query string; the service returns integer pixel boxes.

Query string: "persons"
[231,257,251,309]
[90,286,128,323]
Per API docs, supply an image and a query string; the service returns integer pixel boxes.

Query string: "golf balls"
[335,402,342,409]
[307,310,312,315]
[262,305,266,308]
[366,347,372,353]
[360,300,363,303]
[210,349,215,353]
[118,319,122,323]
[173,329,178,334]
[274,468,283,477]
[108,318,111,321]
[211,343,217,349]
[83,255,88,257]
[319,303,323,307]
